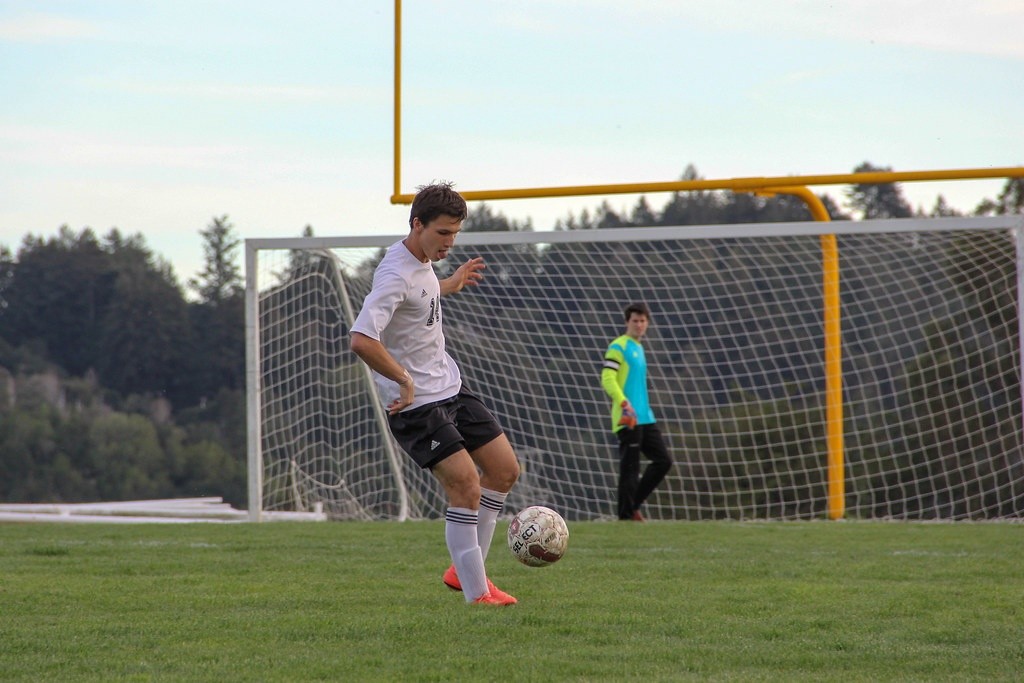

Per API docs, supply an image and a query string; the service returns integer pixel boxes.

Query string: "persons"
[352,183,521,610]
[601,303,672,522]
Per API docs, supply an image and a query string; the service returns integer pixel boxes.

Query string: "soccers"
[506,503,571,567]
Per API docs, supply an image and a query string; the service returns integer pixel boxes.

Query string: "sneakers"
[441,559,517,605]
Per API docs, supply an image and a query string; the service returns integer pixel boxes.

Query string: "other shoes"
[634,509,648,523]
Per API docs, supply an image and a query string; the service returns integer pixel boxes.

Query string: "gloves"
[618,401,636,429]
